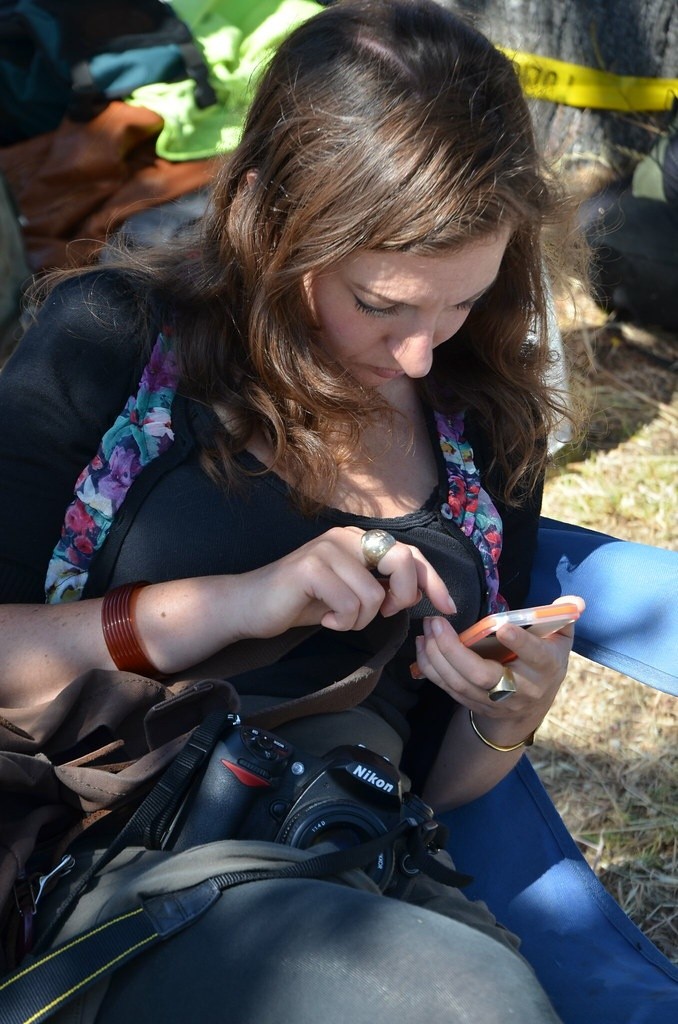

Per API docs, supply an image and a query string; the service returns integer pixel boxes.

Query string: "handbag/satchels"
[0,589,409,975]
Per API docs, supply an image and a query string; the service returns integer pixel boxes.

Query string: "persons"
[0,0,587,1024]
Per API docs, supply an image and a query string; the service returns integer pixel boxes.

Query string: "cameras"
[160,725,438,903]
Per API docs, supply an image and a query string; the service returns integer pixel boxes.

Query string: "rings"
[487,667,515,703]
[361,529,395,563]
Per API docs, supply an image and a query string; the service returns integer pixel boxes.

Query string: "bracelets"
[469,709,541,751]
[101,581,156,673]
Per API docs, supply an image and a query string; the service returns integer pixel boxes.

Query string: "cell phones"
[410,604,581,680]
